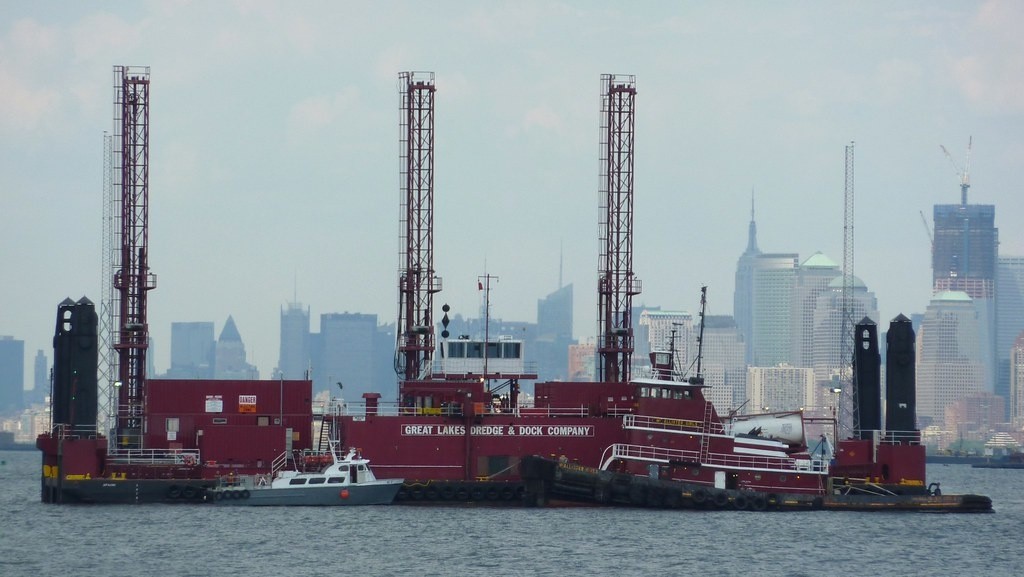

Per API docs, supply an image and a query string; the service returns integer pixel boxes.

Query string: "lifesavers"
[752,492,779,510]
[166,481,197,499]
[397,483,528,501]
[734,494,749,510]
[215,489,250,501]
[692,488,707,504]
[185,456,194,466]
[714,492,728,507]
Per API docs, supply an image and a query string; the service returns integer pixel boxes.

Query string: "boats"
[203,411,406,506]
[518,455,996,513]
[36,65,942,502]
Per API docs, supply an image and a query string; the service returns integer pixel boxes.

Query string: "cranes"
[941,135,974,205]
